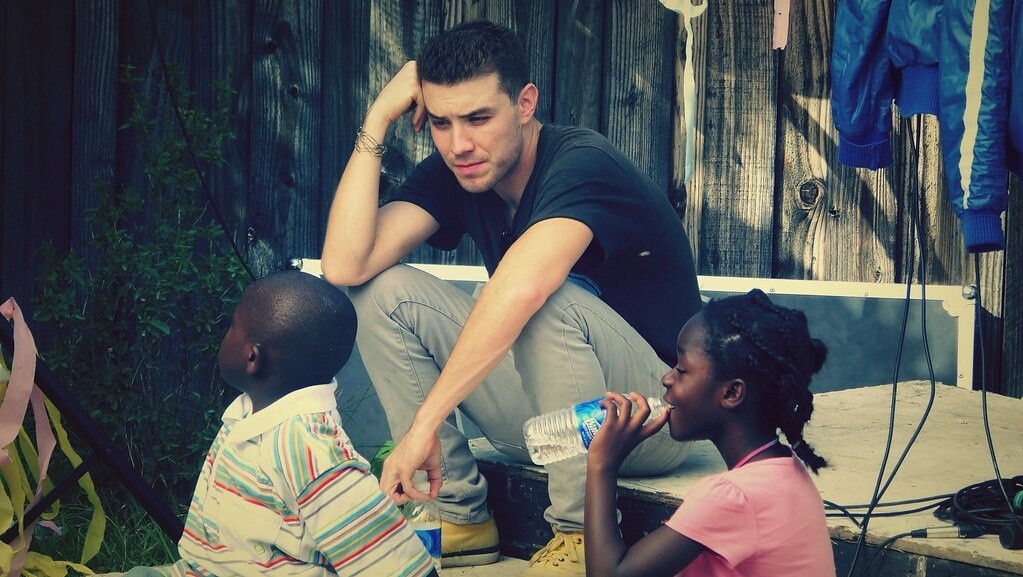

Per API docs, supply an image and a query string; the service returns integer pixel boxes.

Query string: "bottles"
[401,481,441,577]
[523,394,665,465]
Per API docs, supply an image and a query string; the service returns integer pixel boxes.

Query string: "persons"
[118,271,437,577]
[584,288,836,577]
[320,18,703,577]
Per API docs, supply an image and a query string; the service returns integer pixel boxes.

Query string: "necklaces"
[734,437,779,468]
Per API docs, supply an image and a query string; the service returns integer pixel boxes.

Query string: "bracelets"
[354,127,387,157]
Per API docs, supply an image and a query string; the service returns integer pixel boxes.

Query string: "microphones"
[910,524,982,538]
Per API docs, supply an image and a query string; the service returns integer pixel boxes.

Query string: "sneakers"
[407,509,500,566]
[520,523,623,577]
